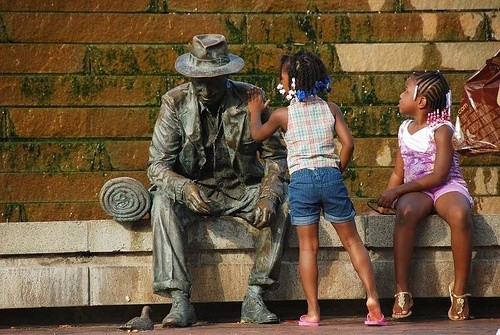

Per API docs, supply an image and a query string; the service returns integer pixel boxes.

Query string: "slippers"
[391,290,414,318]
[299,314,320,326]
[364,313,388,326]
[448,282,474,321]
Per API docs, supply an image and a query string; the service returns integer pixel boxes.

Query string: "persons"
[374,70,474,320]
[147,33,291,326]
[245,51,387,327]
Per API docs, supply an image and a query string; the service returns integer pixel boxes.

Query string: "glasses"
[367,199,398,215]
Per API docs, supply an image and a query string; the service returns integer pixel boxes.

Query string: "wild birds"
[118,305,155,331]
